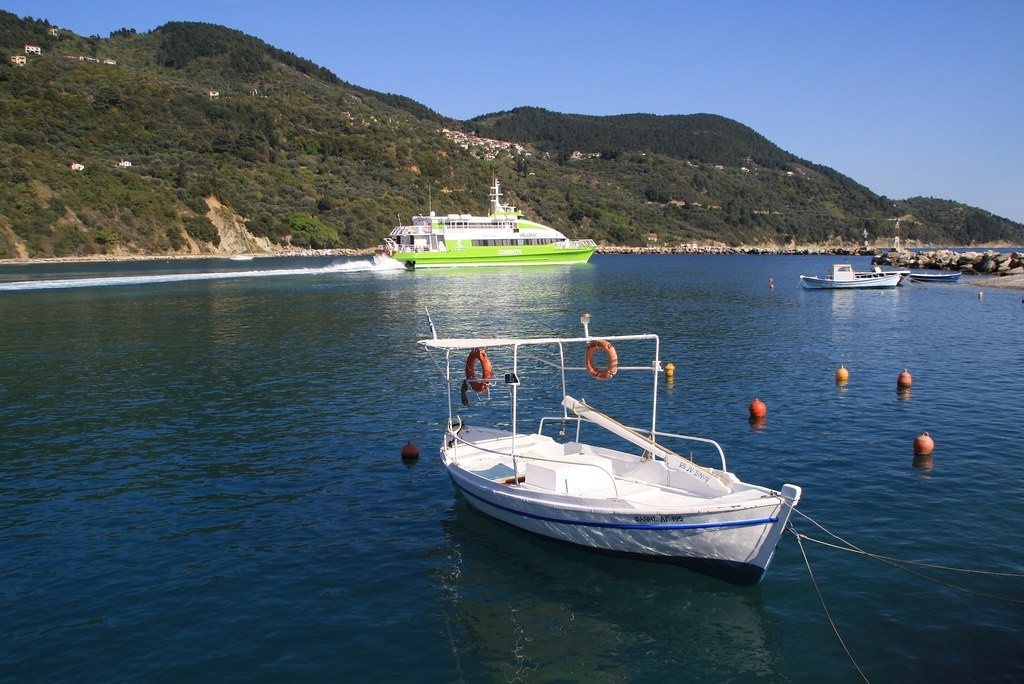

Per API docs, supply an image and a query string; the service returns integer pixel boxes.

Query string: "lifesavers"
[584,338,619,382]
[465,347,492,394]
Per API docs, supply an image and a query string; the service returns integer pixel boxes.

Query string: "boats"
[910,272,962,282]
[417,303,802,570]
[799,264,902,288]
[372,168,599,269]
[825,265,910,285]
